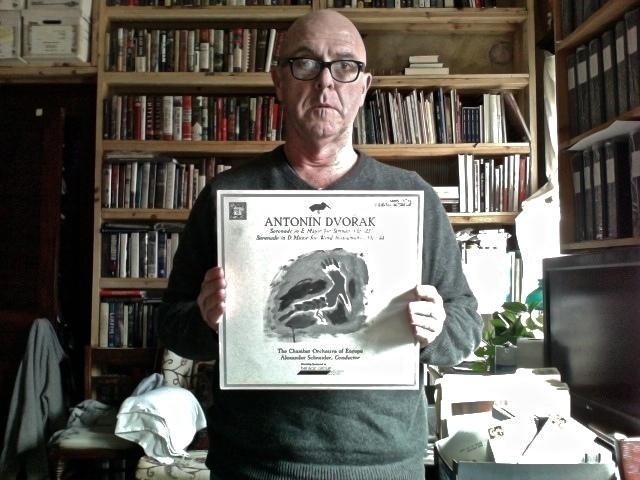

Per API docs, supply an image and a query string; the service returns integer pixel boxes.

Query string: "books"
[106,0,533,9]
[99,290,163,350]
[404,53,450,75]
[430,154,531,212]
[456,228,523,313]
[353,89,530,144]
[103,94,284,141]
[106,27,289,72]
[100,220,186,280]
[101,151,232,209]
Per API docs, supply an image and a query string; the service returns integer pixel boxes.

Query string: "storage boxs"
[435,445,608,480]
[23,10,88,60]
[2,11,22,63]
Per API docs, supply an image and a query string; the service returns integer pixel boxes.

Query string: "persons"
[157,9,483,480]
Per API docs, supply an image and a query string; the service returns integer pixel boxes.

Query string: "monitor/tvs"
[541,245,640,451]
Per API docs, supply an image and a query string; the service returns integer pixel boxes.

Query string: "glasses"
[277,55,367,83]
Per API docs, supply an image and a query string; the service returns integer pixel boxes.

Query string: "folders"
[567,9,640,140]
[572,133,640,242]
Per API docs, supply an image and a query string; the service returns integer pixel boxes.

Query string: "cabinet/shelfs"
[91,0,537,400]
[552,0,639,251]
[0,65,97,341]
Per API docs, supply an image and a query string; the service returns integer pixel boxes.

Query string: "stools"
[56,424,135,480]
